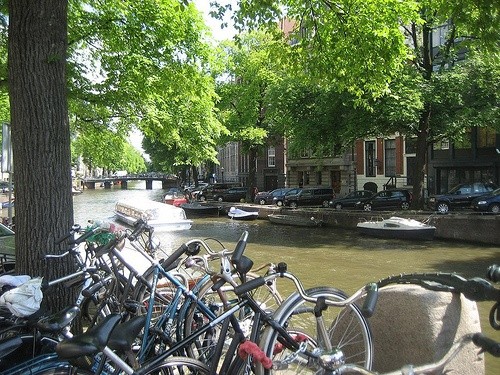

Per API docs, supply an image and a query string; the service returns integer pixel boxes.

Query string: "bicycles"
[0,210,379,375]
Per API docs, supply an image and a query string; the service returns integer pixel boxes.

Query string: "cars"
[354,189,415,212]
[471,187,500,215]
[180,179,337,209]
[0,179,15,193]
[428,180,500,216]
[328,190,379,211]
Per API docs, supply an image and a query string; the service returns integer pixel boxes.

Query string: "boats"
[356,215,436,241]
[227,206,259,221]
